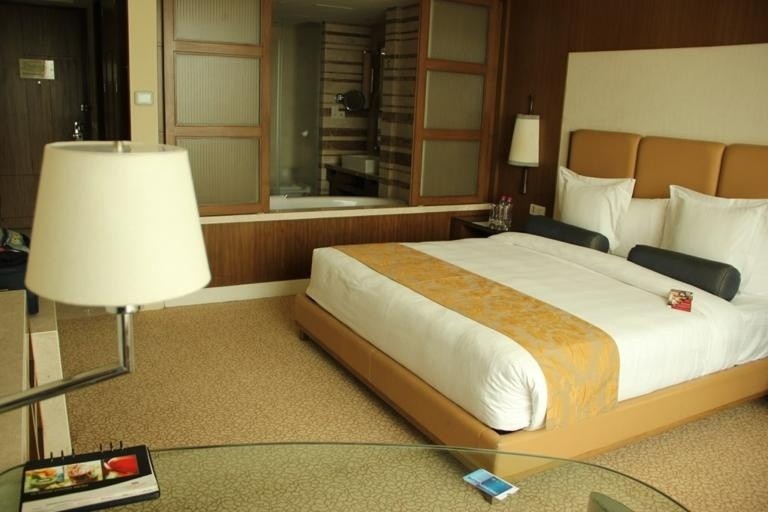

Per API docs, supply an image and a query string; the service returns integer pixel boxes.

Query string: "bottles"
[490,195,515,228]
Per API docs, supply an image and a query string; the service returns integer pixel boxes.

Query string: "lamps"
[0,138,212,415]
[505,91,541,197]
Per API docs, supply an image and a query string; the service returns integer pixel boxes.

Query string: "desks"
[0,441,690,511]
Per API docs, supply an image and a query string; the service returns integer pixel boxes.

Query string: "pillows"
[525,166,767,303]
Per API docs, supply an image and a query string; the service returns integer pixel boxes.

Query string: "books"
[20,440,161,512]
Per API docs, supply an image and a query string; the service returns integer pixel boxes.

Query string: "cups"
[488,219,505,231]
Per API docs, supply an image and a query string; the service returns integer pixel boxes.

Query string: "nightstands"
[449,213,529,241]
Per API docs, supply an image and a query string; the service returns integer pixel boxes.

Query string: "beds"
[289,128,768,505]
